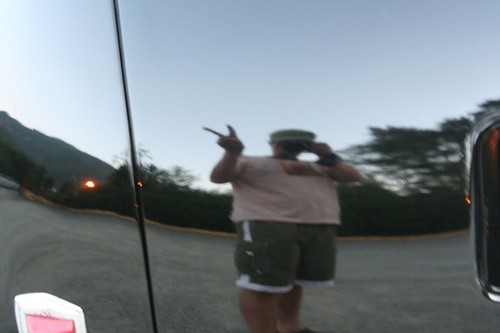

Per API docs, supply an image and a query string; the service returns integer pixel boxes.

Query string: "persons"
[210,125,362,333]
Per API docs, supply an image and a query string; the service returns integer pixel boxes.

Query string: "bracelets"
[323,154,341,166]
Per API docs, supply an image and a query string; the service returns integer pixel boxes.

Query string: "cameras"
[281,140,310,153]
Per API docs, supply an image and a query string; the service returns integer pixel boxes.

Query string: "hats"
[268,130,314,146]
